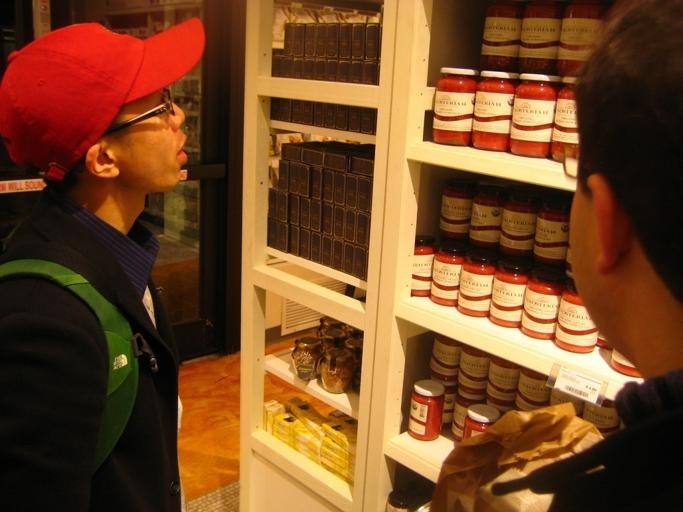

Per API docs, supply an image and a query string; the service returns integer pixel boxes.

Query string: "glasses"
[104,86,175,135]
[561,142,581,177]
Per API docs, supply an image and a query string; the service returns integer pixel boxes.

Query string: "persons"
[0,18,206,512]
[491,0,682,512]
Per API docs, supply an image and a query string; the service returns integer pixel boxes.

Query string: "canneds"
[437,177,576,278]
[407,331,629,445]
[480,1,609,77]
[409,235,649,378]
[430,67,583,162]
[290,315,363,393]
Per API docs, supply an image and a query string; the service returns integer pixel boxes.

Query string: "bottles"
[291,316,365,394]
[429,333,625,442]
[408,379,445,440]
[462,405,500,439]
[431,2,610,166]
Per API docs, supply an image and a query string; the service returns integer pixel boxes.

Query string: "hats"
[0,17,206,182]
